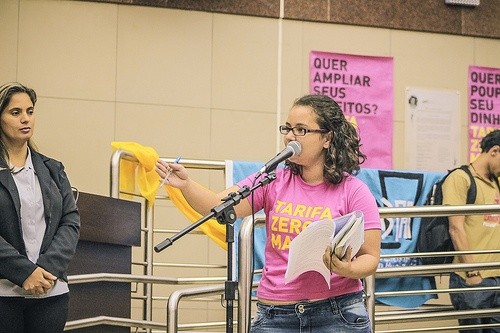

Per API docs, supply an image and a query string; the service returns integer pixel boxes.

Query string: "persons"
[439,129,500,333]
[0,81,81,333]
[154,94,383,333]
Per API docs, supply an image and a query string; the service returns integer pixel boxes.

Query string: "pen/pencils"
[159,153,183,187]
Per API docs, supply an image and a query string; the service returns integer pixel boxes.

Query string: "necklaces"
[470,163,483,179]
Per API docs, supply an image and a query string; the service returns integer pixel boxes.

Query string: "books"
[320,209,364,265]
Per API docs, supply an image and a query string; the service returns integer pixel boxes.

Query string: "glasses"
[279,125,331,136]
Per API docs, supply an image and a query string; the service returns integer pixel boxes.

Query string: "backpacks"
[414,164,500,266]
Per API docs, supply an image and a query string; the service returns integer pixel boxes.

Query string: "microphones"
[255,141,302,179]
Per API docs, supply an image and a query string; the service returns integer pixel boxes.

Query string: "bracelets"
[466,272,481,277]
